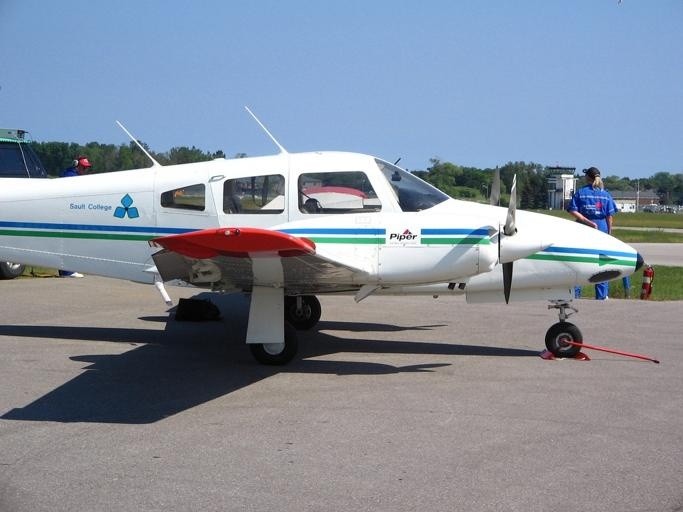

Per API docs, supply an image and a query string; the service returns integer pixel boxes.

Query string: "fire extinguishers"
[641,263,654,299]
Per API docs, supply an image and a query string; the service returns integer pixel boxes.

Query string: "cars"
[644,205,676,214]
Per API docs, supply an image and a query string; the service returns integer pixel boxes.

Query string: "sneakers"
[596,295,609,300]
[58,271,84,279]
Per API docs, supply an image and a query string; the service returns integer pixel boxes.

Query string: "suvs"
[0,127,48,279]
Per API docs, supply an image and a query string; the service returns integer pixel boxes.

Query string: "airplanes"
[0,106,644,366]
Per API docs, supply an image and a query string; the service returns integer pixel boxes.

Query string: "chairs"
[300,198,321,214]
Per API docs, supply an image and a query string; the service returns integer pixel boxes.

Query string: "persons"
[56,156,92,277]
[567,167,618,299]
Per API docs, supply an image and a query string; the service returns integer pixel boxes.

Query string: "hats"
[79,158,92,168]
[582,167,600,179]
